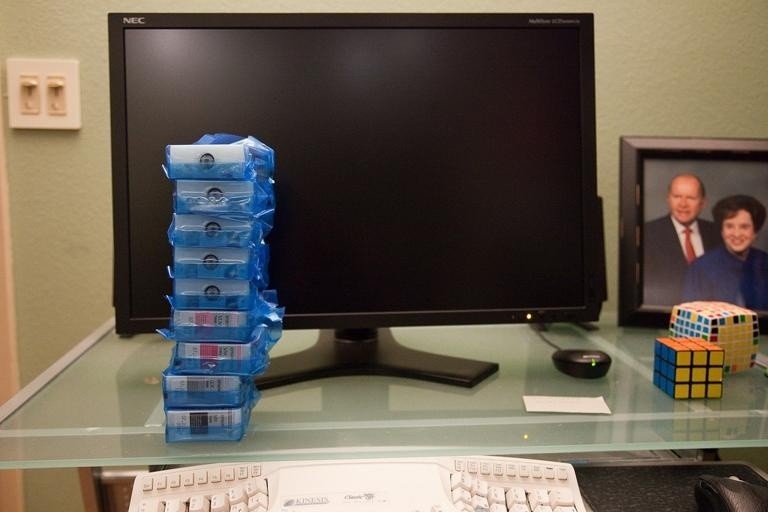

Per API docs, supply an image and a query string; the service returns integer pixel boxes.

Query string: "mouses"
[695,473,768,512]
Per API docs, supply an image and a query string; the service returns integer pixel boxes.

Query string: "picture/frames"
[619,133,768,328]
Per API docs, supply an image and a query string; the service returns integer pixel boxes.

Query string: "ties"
[681,226,696,264]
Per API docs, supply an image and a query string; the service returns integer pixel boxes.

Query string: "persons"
[643,174,717,305]
[681,195,768,310]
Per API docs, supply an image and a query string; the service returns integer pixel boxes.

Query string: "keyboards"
[129,457,591,512]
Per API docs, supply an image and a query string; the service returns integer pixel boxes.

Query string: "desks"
[0,313,768,512]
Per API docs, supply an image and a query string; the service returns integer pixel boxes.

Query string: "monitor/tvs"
[108,12,603,393]
